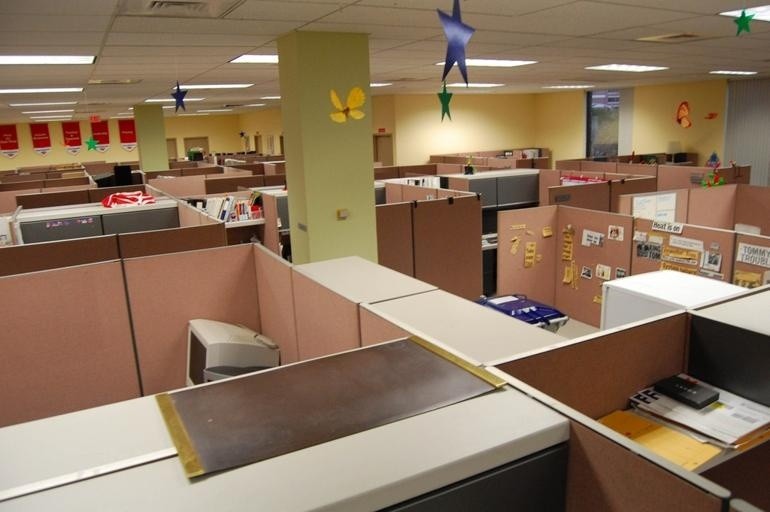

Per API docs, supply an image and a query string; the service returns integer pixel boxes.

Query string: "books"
[630,371,770,454]
[194,195,251,223]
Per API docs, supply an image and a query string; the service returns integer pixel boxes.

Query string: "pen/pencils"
[236,204,246,216]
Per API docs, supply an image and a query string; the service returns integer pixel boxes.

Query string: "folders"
[207,196,235,220]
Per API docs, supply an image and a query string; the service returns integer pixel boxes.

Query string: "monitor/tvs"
[186,319,280,386]
